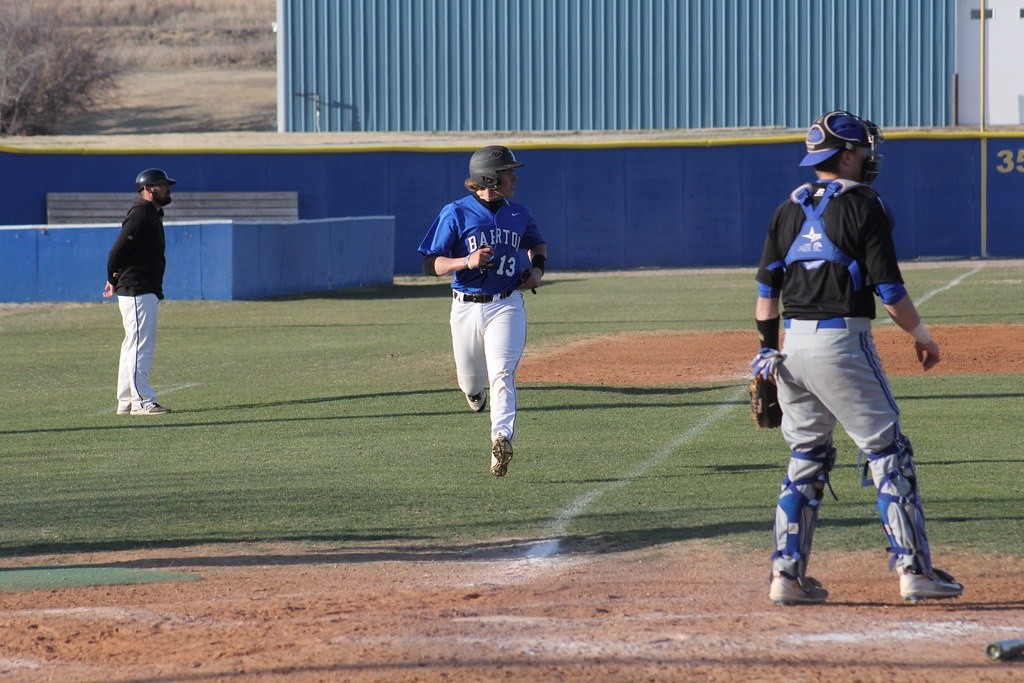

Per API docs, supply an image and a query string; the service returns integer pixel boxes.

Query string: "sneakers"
[117,410,130,414]
[770,572,828,604]
[490,438,513,476]
[131,403,170,415]
[897,567,963,603]
[466,388,487,413]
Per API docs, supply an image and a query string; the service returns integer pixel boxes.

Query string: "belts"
[785,319,847,330]
[454,290,514,302]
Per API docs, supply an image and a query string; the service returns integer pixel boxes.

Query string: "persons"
[103,169,176,414]
[417,146,547,478]
[751,112,963,606]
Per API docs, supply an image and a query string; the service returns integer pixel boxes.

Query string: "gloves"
[750,347,786,379]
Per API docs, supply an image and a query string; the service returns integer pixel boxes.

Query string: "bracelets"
[466,255,471,268]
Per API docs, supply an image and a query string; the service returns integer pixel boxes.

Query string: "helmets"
[136,168,176,192]
[798,115,868,167]
[470,145,525,189]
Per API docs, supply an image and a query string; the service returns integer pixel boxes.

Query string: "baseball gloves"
[749,375,782,429]
